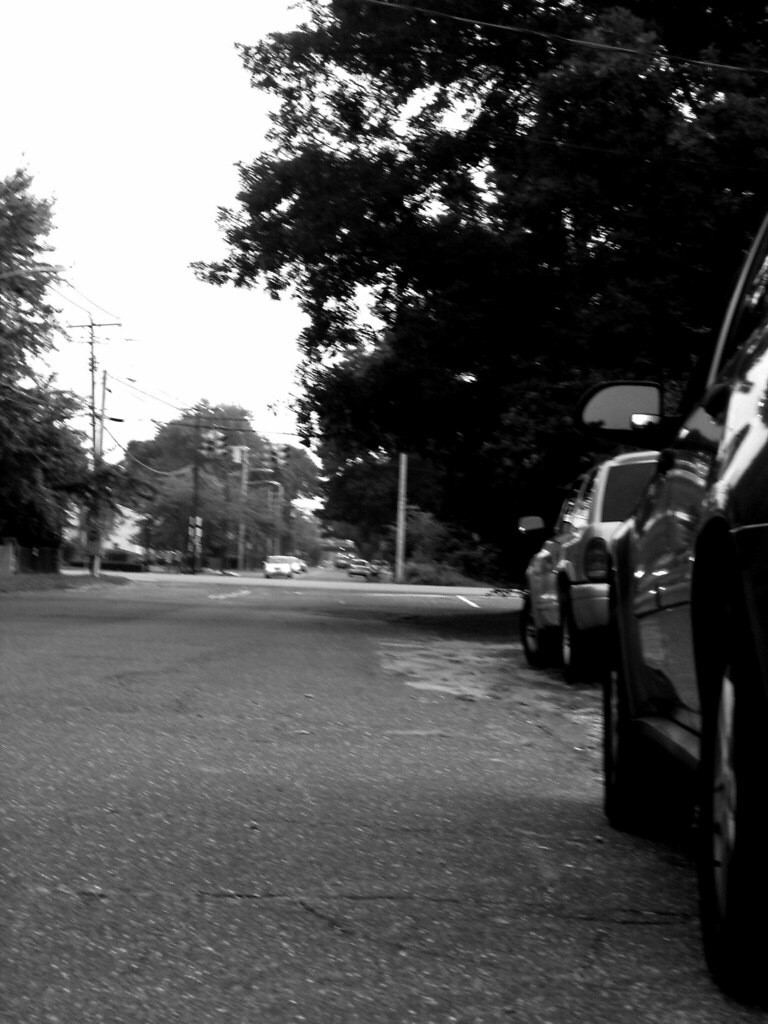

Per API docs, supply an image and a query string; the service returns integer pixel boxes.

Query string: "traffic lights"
[259,446,271,474]
[228,445,241,465]
[213,432,227,461]
[200,432,214,460]
[271,446,277,473]
[279,445,289,473]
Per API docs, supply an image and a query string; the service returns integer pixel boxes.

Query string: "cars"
[332,551,391,579]
[578,207,768,1010]
[517,451,660,683]
[264,556,310,577]
